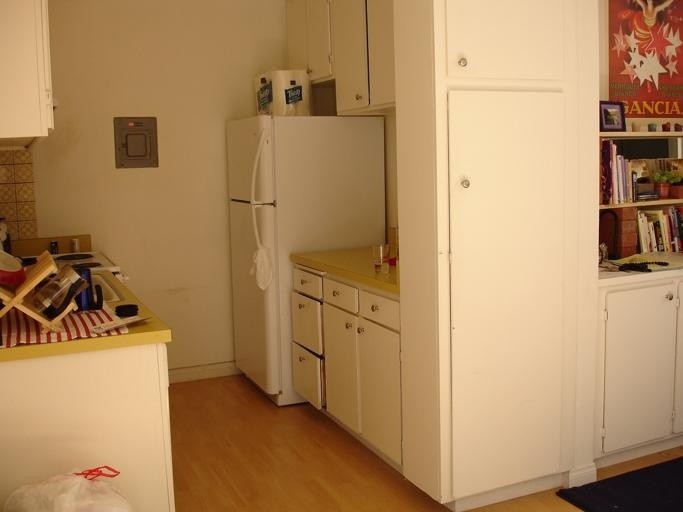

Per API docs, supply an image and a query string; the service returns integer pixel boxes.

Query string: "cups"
[369,241,384,264]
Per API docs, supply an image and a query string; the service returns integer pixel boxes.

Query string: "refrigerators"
[226,115,388,407]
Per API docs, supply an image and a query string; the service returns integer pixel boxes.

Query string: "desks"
[0,270,177,512]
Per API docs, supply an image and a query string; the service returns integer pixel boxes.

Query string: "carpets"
[555,452,683,512]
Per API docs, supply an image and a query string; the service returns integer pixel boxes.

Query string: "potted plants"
[650,168,683,199]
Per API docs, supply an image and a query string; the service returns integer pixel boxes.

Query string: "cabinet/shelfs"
[280,0,392,120]
[390,0,601,511]
[598,118,683,278]
[324,273,402,476]
[289,262,328,413]
[596,281,683,470]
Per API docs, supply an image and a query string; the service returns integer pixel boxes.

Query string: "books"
[600,140,683,260]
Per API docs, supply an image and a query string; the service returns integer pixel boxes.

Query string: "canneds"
[50,240,58,254]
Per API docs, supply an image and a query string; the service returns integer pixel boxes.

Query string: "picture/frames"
[600,100,627,131]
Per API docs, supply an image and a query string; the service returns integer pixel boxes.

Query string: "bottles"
[49,241,59,255]
[30,264,80,314]
